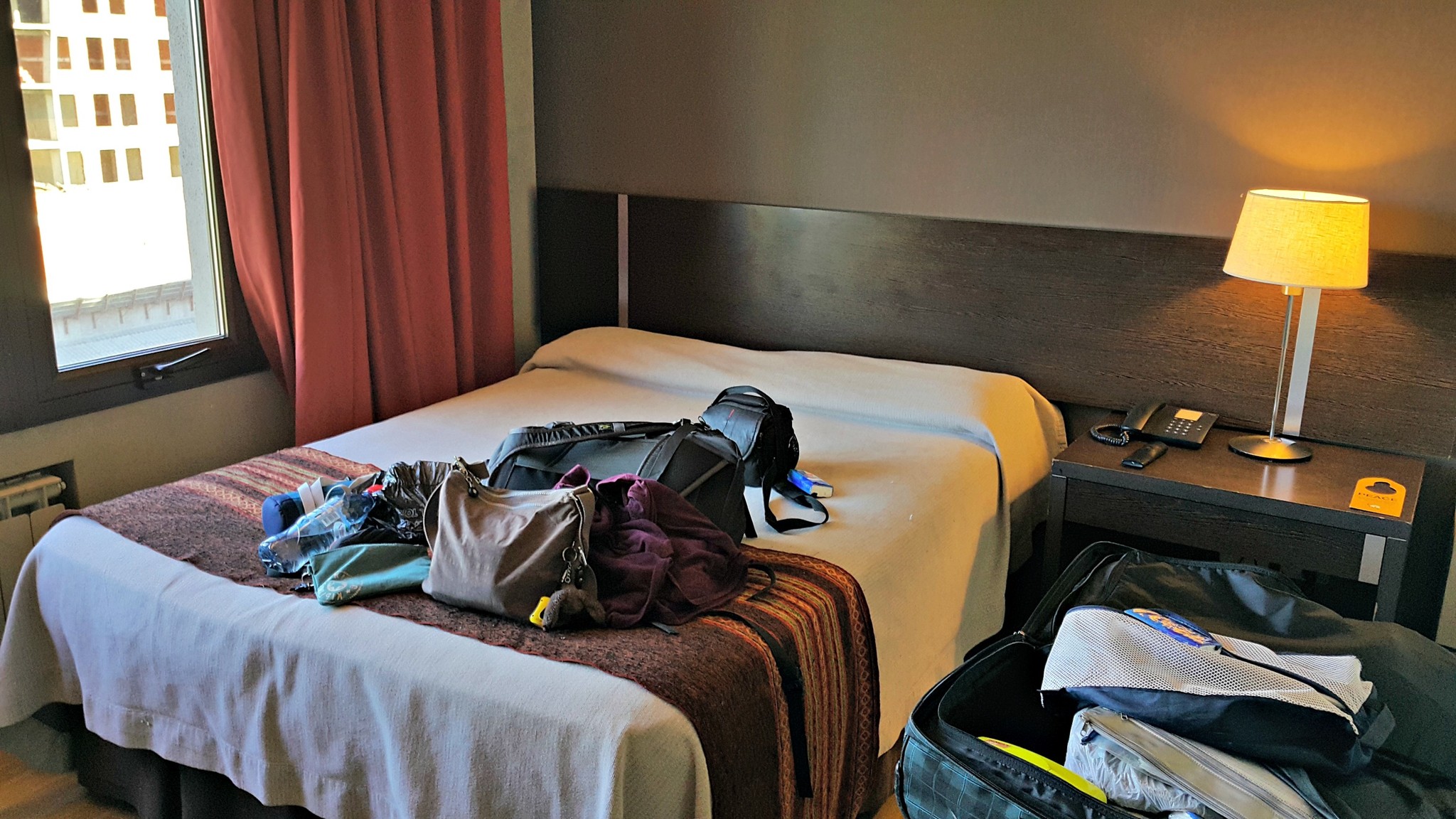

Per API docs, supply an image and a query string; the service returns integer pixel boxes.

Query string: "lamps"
[1219,184,1375,467]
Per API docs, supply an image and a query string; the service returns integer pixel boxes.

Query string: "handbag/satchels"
[420,464,594,628]
[703,386,801,488]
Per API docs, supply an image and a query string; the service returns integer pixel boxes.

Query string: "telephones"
[1090,399,1219,451]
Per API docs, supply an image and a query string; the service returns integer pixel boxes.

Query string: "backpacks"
[490,419,746,551]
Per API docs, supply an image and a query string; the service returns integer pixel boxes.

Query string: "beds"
[5,327,1069,819]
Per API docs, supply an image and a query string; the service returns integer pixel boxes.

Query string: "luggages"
[895,538,1456,818]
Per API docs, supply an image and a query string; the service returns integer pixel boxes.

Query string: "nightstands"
[1045,411,1428,634]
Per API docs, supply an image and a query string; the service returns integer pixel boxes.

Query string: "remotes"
[1121,441,1168,467]
